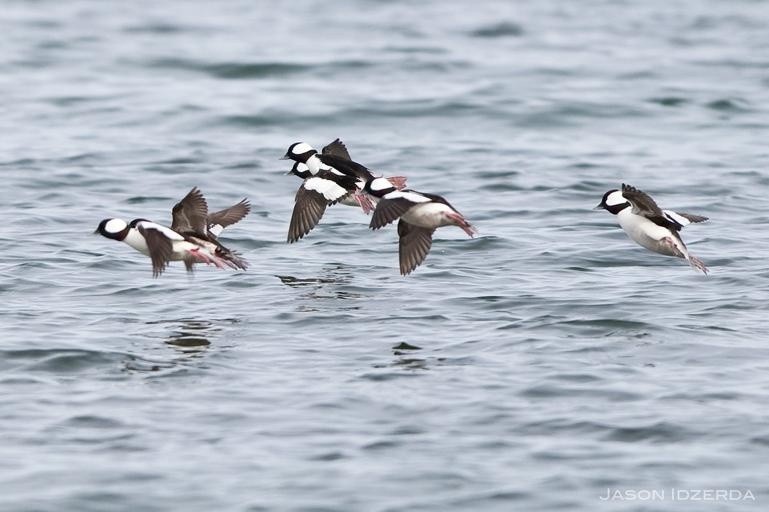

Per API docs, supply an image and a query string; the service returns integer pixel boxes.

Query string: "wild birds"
[592,182,711,276]
[126,186,252,272]
[359,176,475,277]
[278,140,407,244]
[93,217,225,280]
[283,137,376,210]
